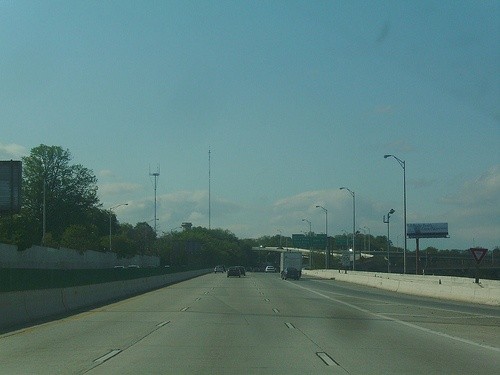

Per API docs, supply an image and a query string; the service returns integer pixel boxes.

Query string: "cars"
[222,265,226,272]
[114,265,126,269]
[127,265,140,269]
[214,266,224,273]
[236,266,246,276]
[265,266,275,273]
[227,266,241,278]
[280,267,299,280]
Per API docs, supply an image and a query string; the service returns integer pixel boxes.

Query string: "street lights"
[383,154,407,275]
[276,230,283,249]
[301,218,312,270]
[109,203,128,252]
[340,187,355,271]
[144,219,159,255]
[315,205,328,270]
